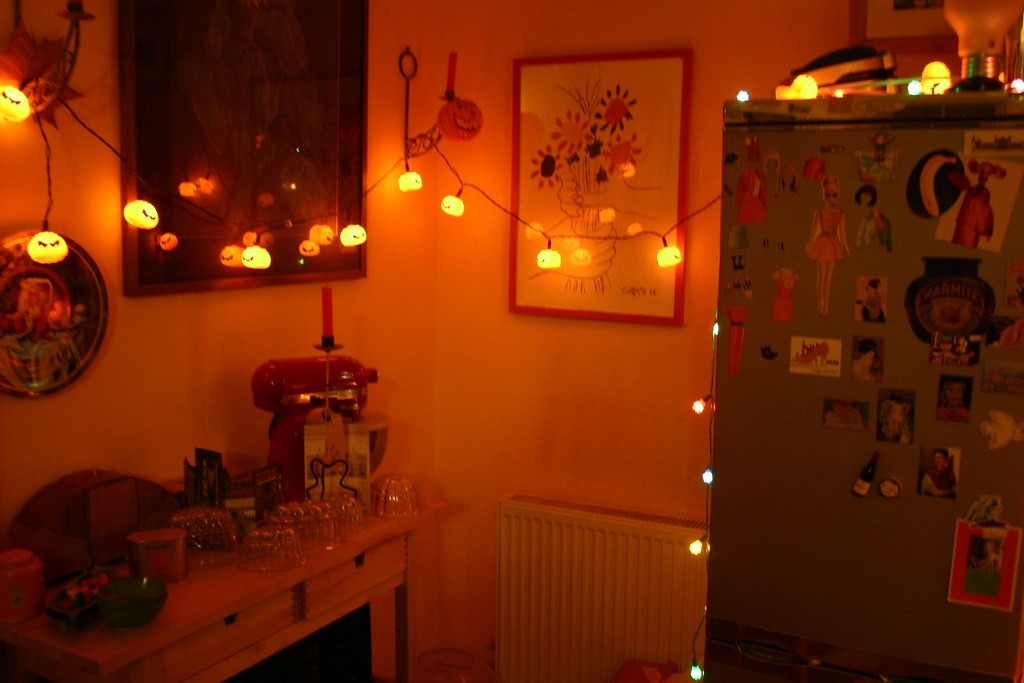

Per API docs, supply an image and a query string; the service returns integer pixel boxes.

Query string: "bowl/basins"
[97,576,168,629]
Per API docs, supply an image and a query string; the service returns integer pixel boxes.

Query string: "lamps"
[944,0,1024,81]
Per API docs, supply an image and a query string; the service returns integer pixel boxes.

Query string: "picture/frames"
[509,46,693,327]
[116,0,371,300]
[866,0,952,40]
[0,228,109,400]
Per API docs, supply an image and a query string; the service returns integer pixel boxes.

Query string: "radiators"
[495,493,707,683]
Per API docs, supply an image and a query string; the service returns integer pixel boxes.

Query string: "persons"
[923,448,956,499]
[938,379,969,423]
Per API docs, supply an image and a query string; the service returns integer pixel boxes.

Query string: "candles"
[446,51,457,90]
[320,281,333,338]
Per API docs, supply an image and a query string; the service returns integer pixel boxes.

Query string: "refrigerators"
[699,96,1024,683]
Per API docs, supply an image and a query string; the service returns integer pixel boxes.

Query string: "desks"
[0,512,420,683]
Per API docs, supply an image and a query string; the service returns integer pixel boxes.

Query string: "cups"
[166,468,422,572]
